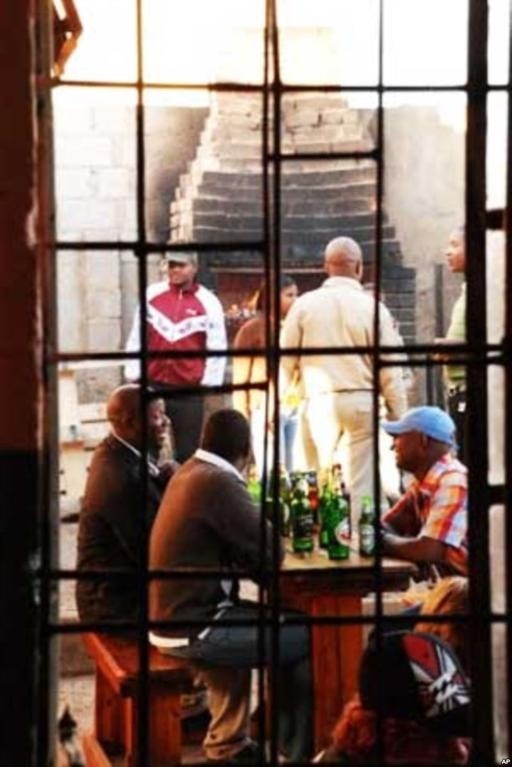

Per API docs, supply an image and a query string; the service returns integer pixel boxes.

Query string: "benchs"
[80,629,240,767]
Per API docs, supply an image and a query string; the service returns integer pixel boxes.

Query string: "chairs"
[360,629,480,766]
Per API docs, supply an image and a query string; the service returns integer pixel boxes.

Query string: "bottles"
[240,462,350,562]
[356,497,387,556]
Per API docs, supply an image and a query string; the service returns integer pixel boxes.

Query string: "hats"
[380,406,456,444]
[161,252,198,264]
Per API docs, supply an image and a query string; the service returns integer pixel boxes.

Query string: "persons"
[123,249,228,461]
[378,407,470,644]
[438,226,469,463]
[280,236,410,516]
[75,383,260,764]
[147,409,316,764]
[319,573,470,766]
[233,273,301,479]
